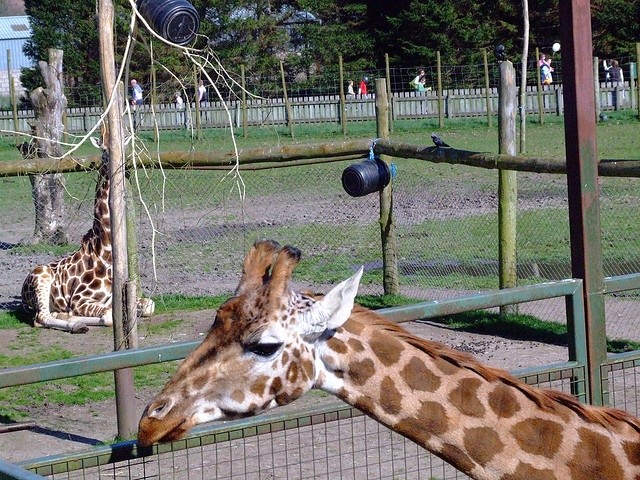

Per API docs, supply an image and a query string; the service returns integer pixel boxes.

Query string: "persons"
[176,92,183,103]
[603,60,612,82]
[417,77,431,91]
[359,77,368,94]
[537,53,554,72]
[131,79,143,105]
[348,81,355,94]
[541,55,553,85]
[198,80,207,107]
[408,69,425,91]
[609,60,624,82]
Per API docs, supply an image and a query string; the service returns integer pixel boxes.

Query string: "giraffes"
[21,121,156,334]
[137,240,640,480]
